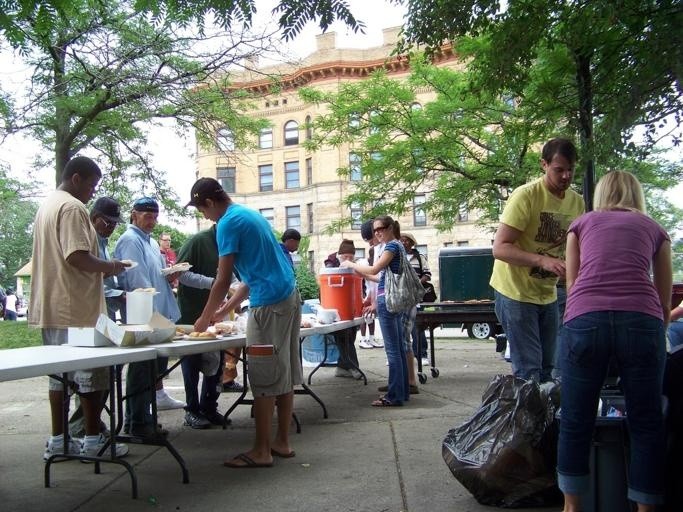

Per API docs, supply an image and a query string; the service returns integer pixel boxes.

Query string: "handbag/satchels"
[386,244,426,315]
[416,252,437,303]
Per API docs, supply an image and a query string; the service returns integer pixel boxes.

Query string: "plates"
[120,260,139,270]
[172,331,185,341]
[130,290,162,296]
[158,262,193,278]
[184,335,224,340]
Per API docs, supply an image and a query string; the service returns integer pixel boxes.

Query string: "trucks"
[438,247,495,339]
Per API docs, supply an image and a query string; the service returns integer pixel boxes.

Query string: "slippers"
[227,454,273,467]
[271,447,296,458]
[371,396,401,408]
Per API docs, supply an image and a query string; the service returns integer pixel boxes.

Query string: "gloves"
[108,260,132,277]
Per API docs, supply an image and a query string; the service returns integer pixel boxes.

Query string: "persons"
[279,230,301,265]
[66,196,126,440]
[2,289,18,321]
[358,277,383,349]
[173,223,237,429]
[28,157,132,463]
[184,178,303,468]
[555,169,673,512]
[490,140,584,384]
[397,234,437,373]
[213,299,249,392]
[158,233,179,297]
[370,216,425,407]
[324,240,364,379]
[362,221,418,392]
[113,197,181,440]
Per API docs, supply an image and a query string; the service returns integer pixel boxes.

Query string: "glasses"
[158,238,170,242]
[99,217,120,228]
[372,225,387,233]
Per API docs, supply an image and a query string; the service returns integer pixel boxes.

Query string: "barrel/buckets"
[319,267,356,321]
[354,269,363,318]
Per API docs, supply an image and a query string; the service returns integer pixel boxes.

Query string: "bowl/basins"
[317,309,339,323]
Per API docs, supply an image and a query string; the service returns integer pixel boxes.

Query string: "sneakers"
[46,436,91,459]
[335,366,350,377]
[125,419,162,435]
[359,337,373,349]
[128,423,167,440]
[223,380,245,393]
[157,394,183,409]
[419,358,431,366]
[350,367,362,379]
[184,411,211,427]
[378,385,390,391]
[199,409,232,423]
[369,336,384,347]
[406,384,419,393]
[81,436,131,460]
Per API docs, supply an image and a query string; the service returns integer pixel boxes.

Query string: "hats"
[185,179,224,209]
[339,239,355,255]
[134,199,160,212]
[283,230,304,239]
[93,197,124,222]
[399,231,418,244]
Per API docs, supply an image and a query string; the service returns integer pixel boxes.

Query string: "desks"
[250,313,373,417]
[1,345,156,511]
[413,310,498,384]
[95,333,301,477]
